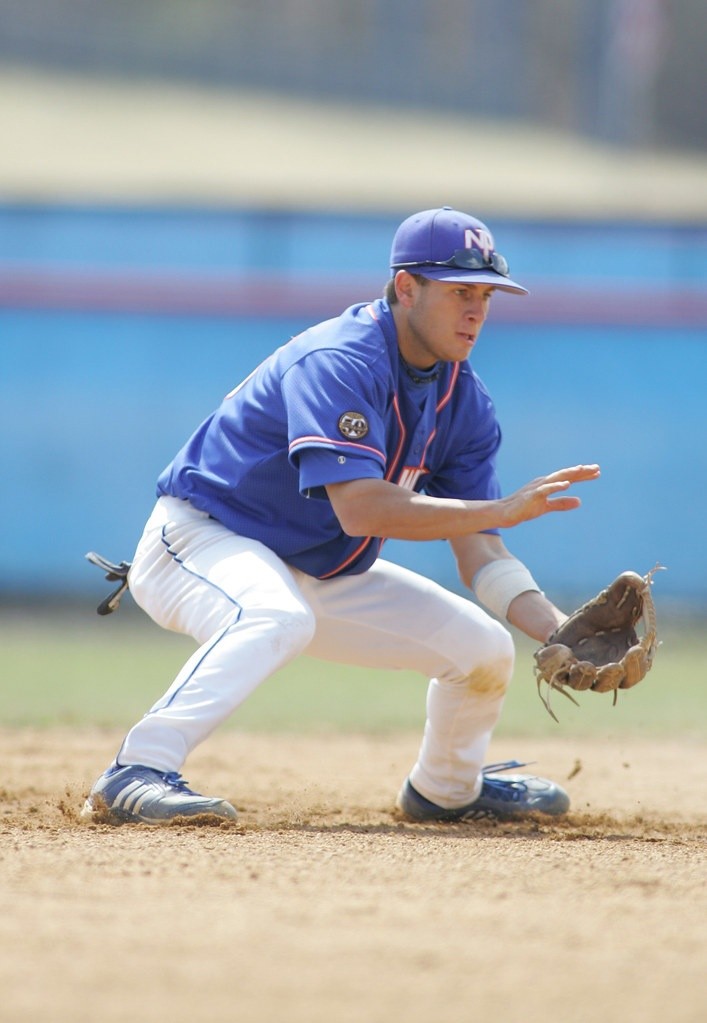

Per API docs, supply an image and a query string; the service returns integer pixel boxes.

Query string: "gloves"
[83,551,132,616]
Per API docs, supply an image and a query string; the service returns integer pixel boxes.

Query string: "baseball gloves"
[532,568,660,693]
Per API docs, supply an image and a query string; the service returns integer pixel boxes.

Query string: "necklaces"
[398,345,443,384]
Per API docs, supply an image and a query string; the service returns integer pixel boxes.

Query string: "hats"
[389,206,531,296]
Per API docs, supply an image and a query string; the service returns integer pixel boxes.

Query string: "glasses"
[389,247,511,279]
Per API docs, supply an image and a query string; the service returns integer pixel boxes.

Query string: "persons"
[83,205,569,828]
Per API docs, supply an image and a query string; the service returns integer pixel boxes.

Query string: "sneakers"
[394,759,572,827]
[78,758,239,828]
